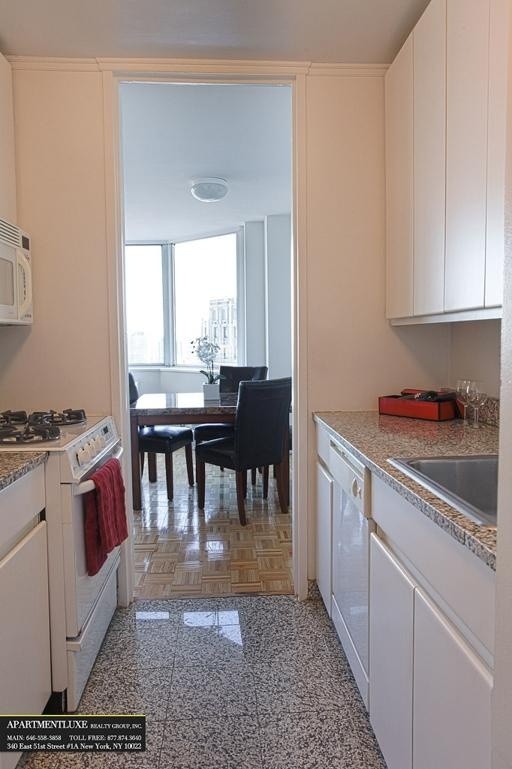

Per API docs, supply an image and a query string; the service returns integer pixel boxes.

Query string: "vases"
[203,383,220,401]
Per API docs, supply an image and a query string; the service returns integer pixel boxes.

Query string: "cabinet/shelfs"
[381,0,512,327]
[367,469,497,769]
[0,461,57,769]
[312,412,368,712]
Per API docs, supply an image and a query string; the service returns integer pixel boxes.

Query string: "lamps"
[190,176,230,203]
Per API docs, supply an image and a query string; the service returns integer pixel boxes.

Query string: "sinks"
[385,454,497,528]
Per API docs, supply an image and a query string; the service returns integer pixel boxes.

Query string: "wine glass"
[453,378,488,431]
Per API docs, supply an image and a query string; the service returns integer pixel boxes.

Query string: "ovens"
[59,416,121,712]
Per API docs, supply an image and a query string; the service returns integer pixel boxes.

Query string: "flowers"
[190,336,227,384]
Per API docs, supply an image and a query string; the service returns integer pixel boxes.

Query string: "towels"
[83,458,130,578]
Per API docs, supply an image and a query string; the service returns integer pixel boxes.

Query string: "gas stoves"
[0,408,107,451]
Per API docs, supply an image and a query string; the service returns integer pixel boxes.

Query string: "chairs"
[128,365,292,527]
